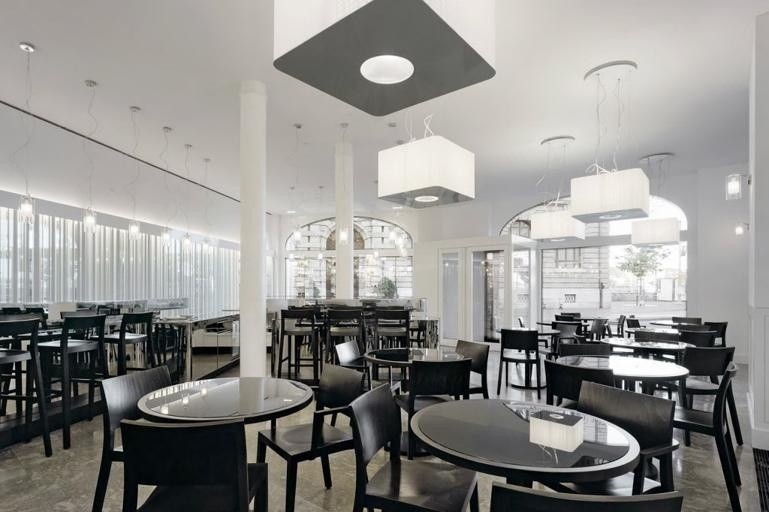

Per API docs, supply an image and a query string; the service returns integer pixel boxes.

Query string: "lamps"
[629,151,681,247]
[569,61,650,223]
[735,221,751,237]
[531,137,586,246]
[271,1,498,117]
[724,172,751,201]
[377,114,477,210]
